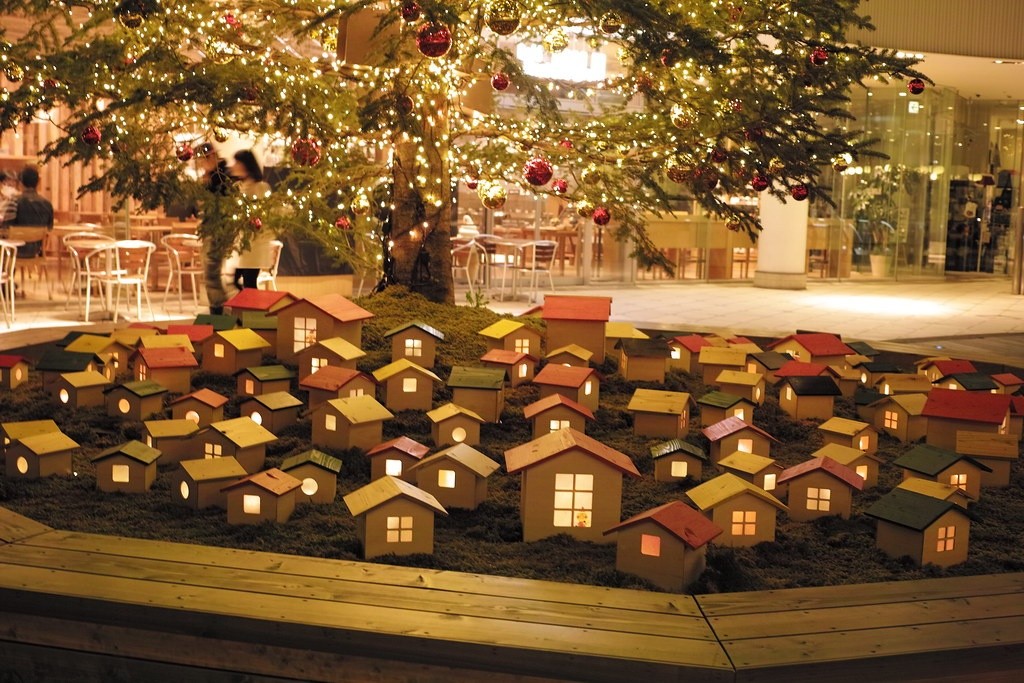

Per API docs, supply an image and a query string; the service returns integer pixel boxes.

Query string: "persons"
[10,167,54,256]
[0,168,17,224]
[196,143,233,313]
[231,149,275,292]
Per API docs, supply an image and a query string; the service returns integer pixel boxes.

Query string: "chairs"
[0,214,756,328]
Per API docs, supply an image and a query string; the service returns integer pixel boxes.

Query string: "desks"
[172,222,207,270]
[131,214,157,226]
[66,239,136,321]
[130,225,179,292]
[53,226,103,233]
[484,237,541,304]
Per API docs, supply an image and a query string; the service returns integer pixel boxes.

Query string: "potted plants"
[849,167,920,278]
